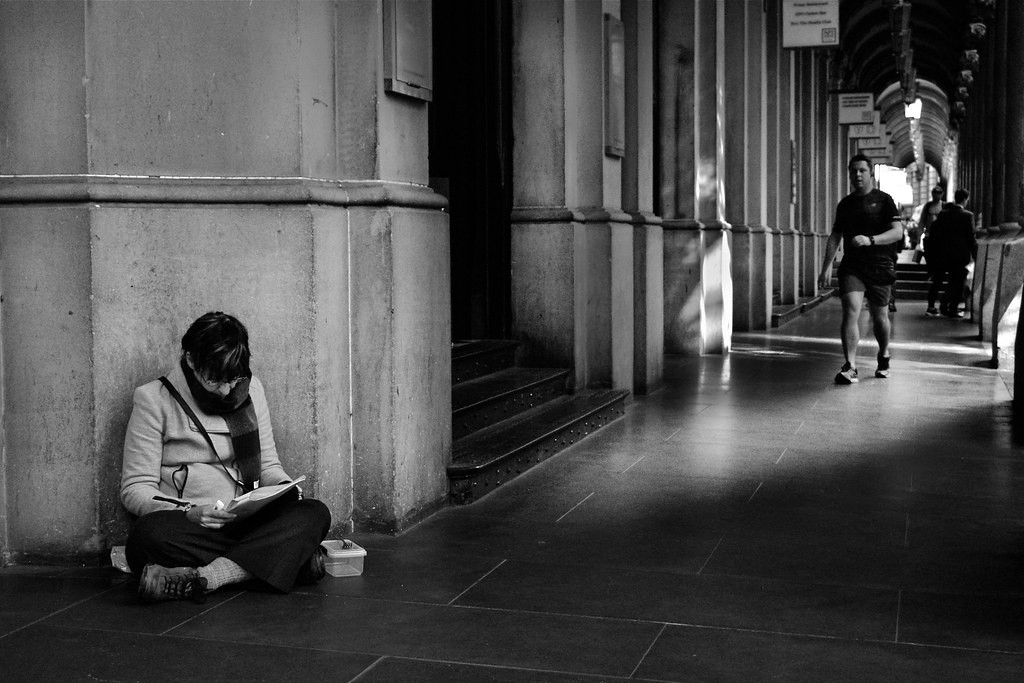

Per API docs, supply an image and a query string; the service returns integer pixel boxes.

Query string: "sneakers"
[925,308,940,316]
[875,351,892,378]
[139,563,213,605]
[834,361,859,383]
[298,548,325,584]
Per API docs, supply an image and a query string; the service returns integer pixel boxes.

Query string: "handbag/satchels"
[912,244,924,263]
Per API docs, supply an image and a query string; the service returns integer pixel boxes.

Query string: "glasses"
[200,369,247,386]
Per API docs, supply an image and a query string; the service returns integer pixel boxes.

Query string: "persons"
[917,186,978,319]
[818,154,903,384]
[865,230,905,312]
[118,311,332,603]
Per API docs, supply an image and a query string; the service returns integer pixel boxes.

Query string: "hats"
[933,186,943,192]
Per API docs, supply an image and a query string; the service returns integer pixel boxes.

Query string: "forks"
[335,533,353,550]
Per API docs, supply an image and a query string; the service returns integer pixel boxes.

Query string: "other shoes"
[889,304,896,312]
[948,312,964,318]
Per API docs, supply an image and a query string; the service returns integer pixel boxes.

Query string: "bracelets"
[868,236,875,246]
